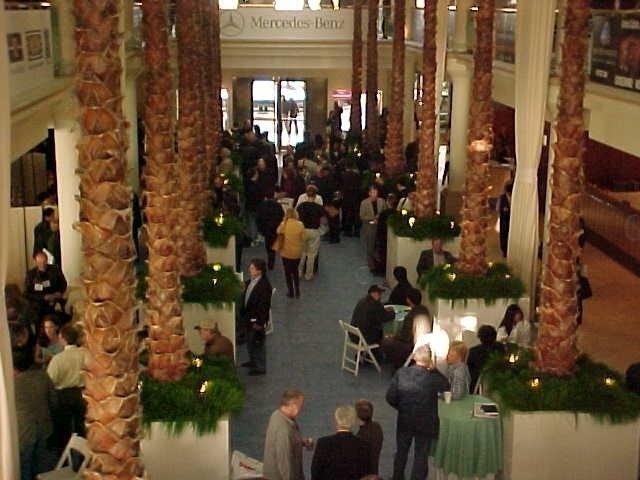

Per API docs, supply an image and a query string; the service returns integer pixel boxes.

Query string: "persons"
[260,387,311,480]
[4,171,89,454]
[355,396,387,479]
[382,343,451,480]
[442,140,450,185]
[211,119,329,297]
[349,237,527,401]
[323,99,420,275]
[195,320,233,365]
[576,212,587,328]
[499,184,514,258]
[241,258,271,376]
[280,95,299,136]
[308,403,372,480]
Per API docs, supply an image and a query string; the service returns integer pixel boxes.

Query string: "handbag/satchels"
[272,233,284,254]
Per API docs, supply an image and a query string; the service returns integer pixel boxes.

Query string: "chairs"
[336,320,382,377]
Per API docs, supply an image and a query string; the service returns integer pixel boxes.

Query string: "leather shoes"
[248,370,266,376]
[241,361,251,367]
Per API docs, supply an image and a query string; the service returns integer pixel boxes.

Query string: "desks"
[424,392,505,480]
[383,303,413,338]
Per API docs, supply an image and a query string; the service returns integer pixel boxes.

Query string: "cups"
[444,392,452,403]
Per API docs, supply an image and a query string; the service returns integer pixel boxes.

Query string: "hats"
[193,318,218,330]
[368,284,386,293]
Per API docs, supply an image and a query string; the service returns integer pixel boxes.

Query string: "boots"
[286,288,294,298]
[295,288,300,297]
